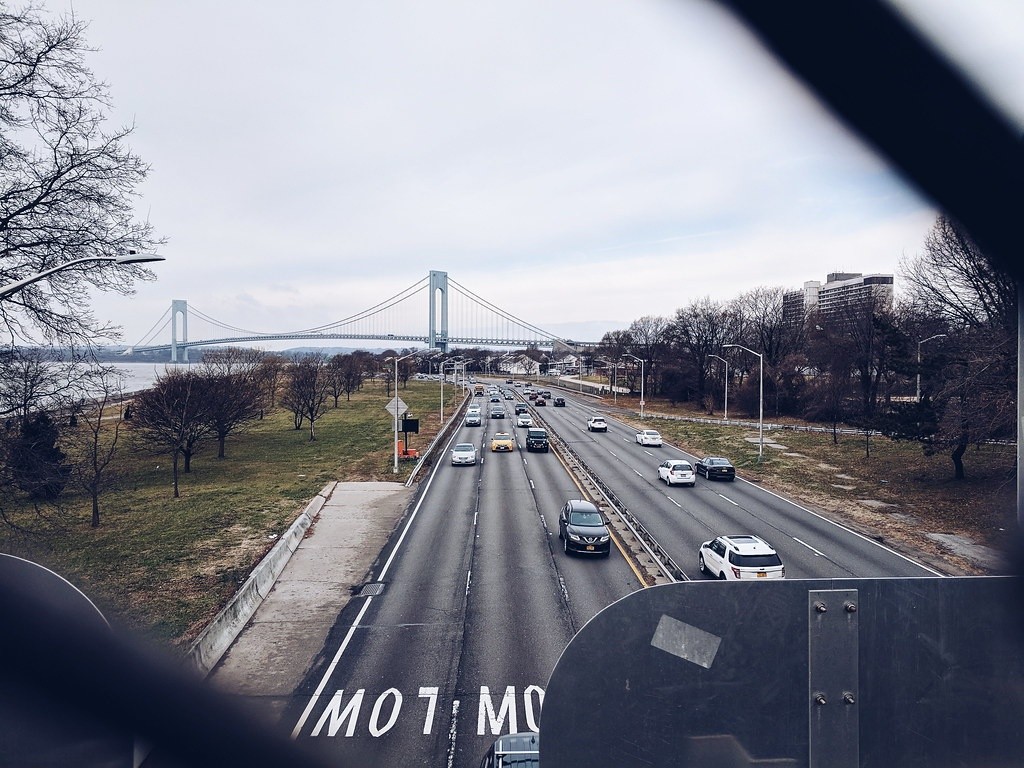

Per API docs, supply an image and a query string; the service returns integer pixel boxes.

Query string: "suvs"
[526,428,549,453]
[559,500,611,559]
[700,534,786,580]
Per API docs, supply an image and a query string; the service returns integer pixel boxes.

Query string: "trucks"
[547,369,560,376]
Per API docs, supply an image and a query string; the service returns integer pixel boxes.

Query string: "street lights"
[438,354,464,425]
[393,346,441,475]
[454,358,475,408]
[708,353,728,420]
[723,343,764,459]
[622,354,645,421]
[1,251,165,310]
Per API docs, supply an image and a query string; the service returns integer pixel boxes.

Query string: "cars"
[658,460,695,487]
[468,404,482,413]
[515,403,528,415]
[636,430,662,448]
[586,416,608,431]
[695,457,735,481]
[414,372,444,380]
[491,406,505,419]
[517,413,533,427]
[470,378,477,384]
[490,432,514,452]
[474,378,566,407]
[465,410,481,427]
[451,444,478,466]
